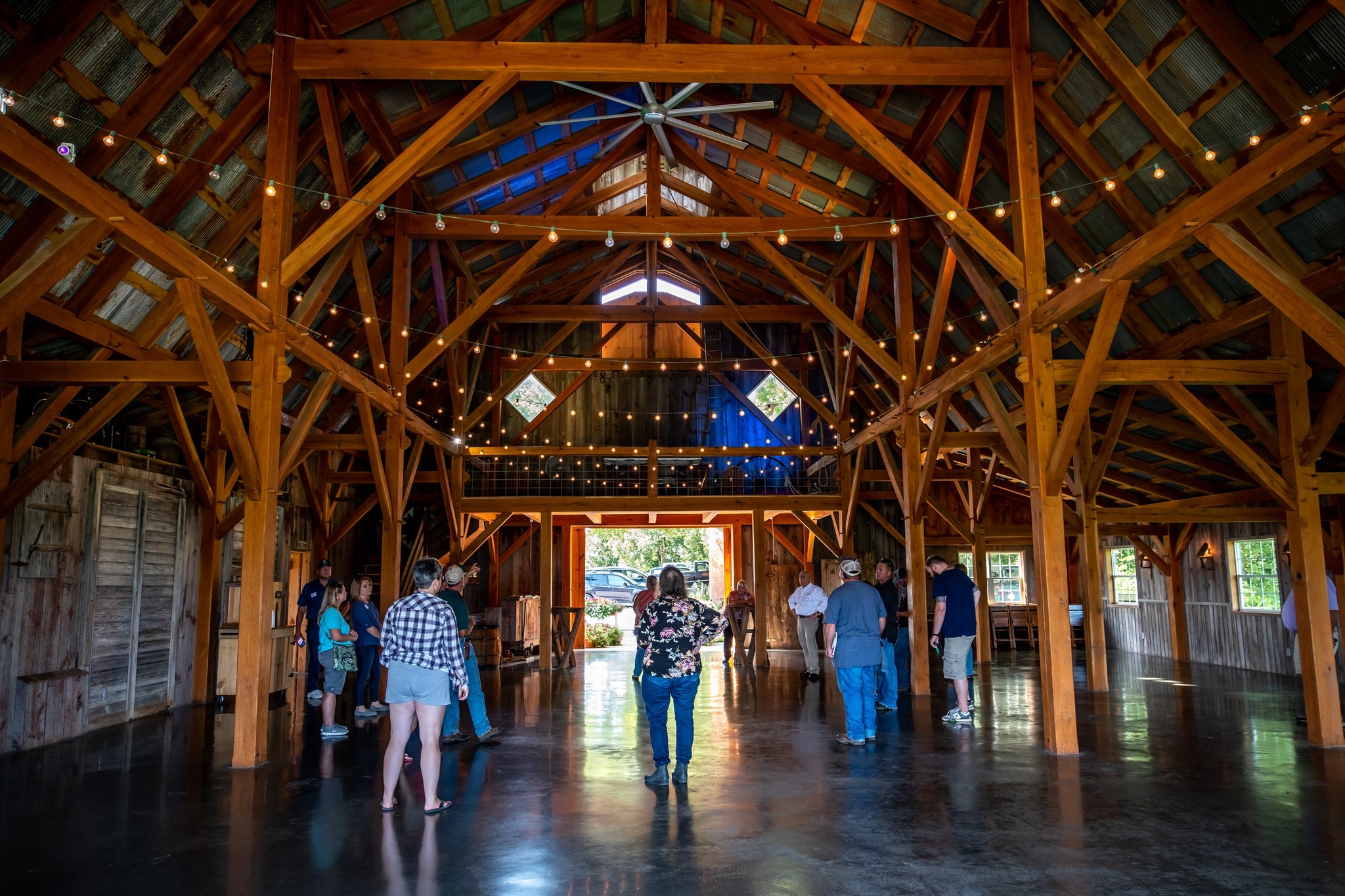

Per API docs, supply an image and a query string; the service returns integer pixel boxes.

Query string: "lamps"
[1196,542,1212,557]
[1282,538,1291,553]
[1137,553,1145,559]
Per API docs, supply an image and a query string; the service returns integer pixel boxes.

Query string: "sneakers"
[955,700,975,711]
[321,722,349,736]
[941,706,972,722]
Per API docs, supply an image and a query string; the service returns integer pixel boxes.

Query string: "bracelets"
[816,612,819,614]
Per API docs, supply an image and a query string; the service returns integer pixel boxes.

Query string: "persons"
[632,575,661,680]
[872,558,910,710]
[722,580,755,663]
[822,556,887,746]
[1278,546,1340,730]
[636,564,729,785]
[925,555,981,722]
[380,555,469,813]
[435,562,504,743]
[293,559,390,736]
[788,571,828,679]
[403,713,444,762]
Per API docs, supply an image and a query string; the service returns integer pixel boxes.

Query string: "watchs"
[932,632,939,636]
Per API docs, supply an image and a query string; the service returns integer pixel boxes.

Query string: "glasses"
[320,567,362,597]
[880,557,891,565]
[799,575,810,579]
[436,575,444,580]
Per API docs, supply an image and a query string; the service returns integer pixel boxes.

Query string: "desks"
[988,603,1036,647]
[726,603,771,668]
[550,605,587,669]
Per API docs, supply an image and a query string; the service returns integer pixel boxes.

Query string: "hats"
[444,565,467,585]
[838,556,861,576]
[893,567,907,584]
[317,559,332,569]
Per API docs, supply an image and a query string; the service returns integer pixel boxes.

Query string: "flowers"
[584,595,624,648]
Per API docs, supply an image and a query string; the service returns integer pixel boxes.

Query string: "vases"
[584,639,595,648]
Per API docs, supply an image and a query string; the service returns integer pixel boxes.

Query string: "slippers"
[380,798,397,811]
[424,800,452,813]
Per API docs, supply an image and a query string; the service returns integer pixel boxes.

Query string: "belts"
[465,638,471,642]
[797,615,811,618]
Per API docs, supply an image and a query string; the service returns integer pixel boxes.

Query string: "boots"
[644,763,688,785]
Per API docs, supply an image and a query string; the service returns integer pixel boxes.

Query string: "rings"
[698,648,699,650]
[930,644,931,645]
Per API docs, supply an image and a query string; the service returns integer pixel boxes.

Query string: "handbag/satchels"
[333,644,358,672]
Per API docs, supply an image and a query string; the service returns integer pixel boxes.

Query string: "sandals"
[403,748,446,761]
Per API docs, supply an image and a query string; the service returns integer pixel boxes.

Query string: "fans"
[532,80,778,171]
[545,455,569,493]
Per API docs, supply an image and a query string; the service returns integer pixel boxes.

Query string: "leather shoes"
[836,733,876,746]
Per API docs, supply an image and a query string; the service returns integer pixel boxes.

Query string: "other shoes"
[945,674,974,687]
[898,685,910,691]
[632,674,638,680]
[307,689,323,699]
[355,705,389,716]
[722,657,729,662]
[798,671,821,679]
[478,725,503,743]
[873,699,888,709]
[442,731,470,743]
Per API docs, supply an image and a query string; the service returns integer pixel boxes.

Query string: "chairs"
[507,472,528,496]
[688,467,710,494]
[988,603,1086,649]
[722,465,747,495]
